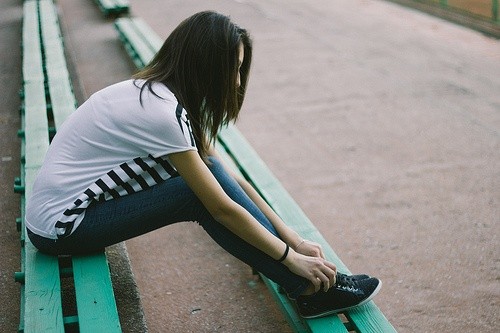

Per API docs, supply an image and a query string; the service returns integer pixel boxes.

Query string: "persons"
[24,12,382,319]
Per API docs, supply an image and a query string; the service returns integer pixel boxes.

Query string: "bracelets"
[295,240,308,250]
[278,245,289,261]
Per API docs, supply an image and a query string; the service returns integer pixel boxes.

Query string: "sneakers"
[286,271,382,319]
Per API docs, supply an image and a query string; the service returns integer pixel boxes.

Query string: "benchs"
[114,17,400,333]
[14,0,123,333]
[92,0,130,18]
